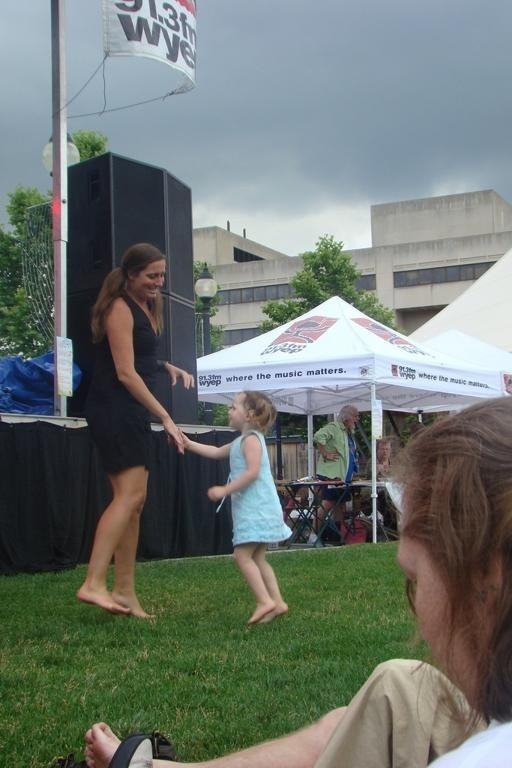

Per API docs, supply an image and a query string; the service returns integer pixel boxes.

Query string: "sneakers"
[307,534,322,547]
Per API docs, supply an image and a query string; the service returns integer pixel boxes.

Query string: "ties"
[345,433,356,483]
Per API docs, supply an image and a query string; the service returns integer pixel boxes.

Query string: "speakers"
[66,151,196,306]
[67,291,204,425]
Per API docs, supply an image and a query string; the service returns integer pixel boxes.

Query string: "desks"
[280,476,405,549]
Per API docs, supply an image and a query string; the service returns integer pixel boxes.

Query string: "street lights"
[195,262,218,425]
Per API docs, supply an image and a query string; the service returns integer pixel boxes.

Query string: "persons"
[75,244,195,618]
[84,396,512,768]
[307,405,400,548]
[176,390,288,625]
